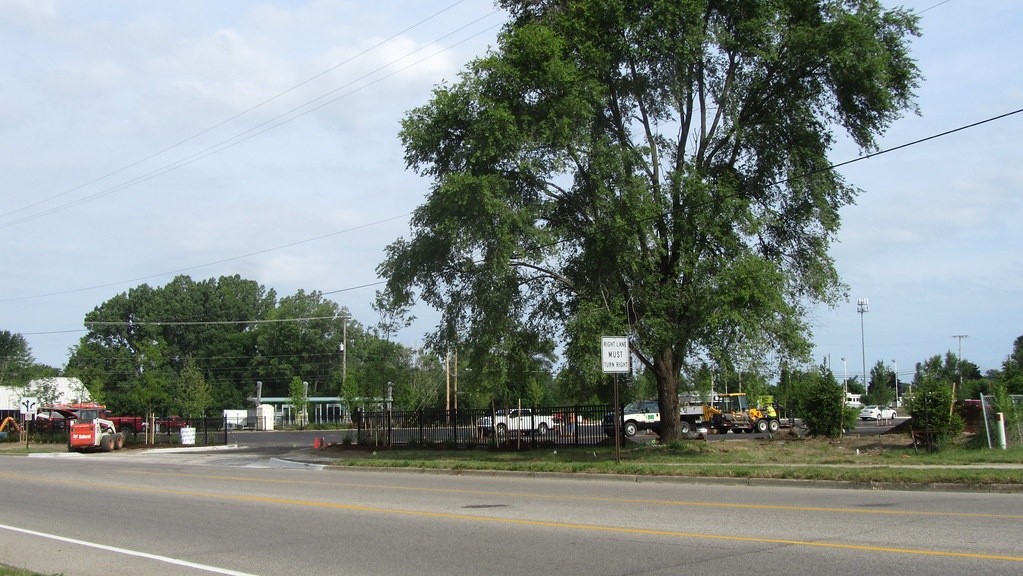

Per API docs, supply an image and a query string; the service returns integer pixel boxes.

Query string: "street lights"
[856,297,871,397]
[892,359,898,408]
[841,357,848,394]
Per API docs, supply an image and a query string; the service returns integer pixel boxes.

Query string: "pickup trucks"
[476,407,555,439]
[598,400,703,436]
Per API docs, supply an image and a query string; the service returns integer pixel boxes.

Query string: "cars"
[859,405,897,421]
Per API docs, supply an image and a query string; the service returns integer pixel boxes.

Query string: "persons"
[84,412,89,419]
[551,409,576,438]
[741,407,752,424]
[764,404,776,419]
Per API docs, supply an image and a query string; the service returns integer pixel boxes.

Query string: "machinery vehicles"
[65,408,124,453]
[703,393,780,433]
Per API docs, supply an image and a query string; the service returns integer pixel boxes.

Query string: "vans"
[845,397,864,407]
